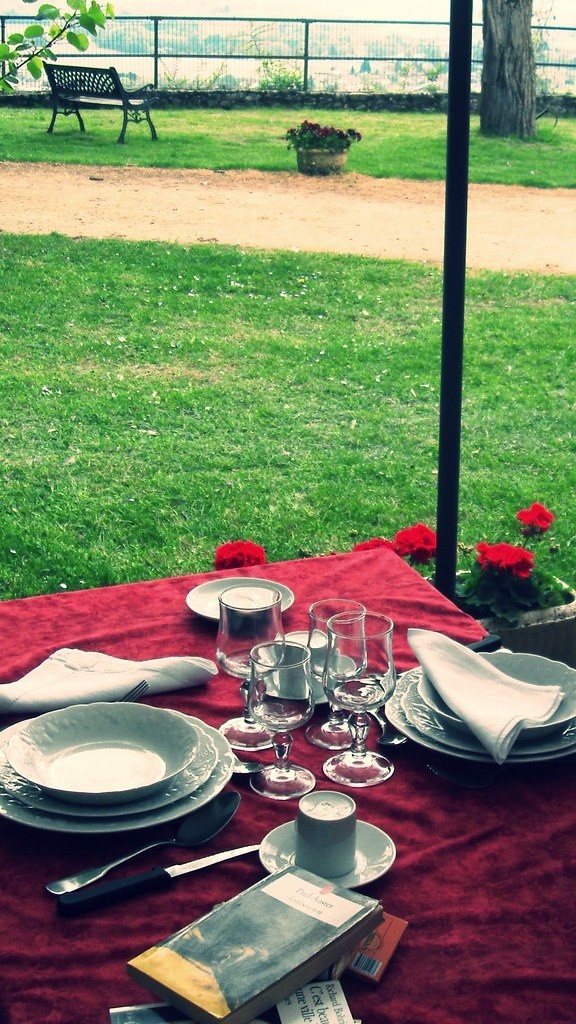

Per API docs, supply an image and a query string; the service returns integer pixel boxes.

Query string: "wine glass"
[305,598,366,752]
[246,639,316,801]
[214,582,286,752]
[322,610,397,788]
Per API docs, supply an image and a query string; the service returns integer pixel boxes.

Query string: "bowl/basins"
[415,652,576,742]
[6,701,200,807]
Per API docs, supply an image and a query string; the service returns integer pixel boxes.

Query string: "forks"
[120,680,149,704]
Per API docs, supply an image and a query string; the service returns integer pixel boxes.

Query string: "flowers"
[214,499,576,625]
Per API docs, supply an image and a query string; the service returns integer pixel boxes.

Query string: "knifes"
[55,843,260,917]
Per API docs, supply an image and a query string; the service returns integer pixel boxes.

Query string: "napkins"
[406,628,566,765]
[0,646,219,714]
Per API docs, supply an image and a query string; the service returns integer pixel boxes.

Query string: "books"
[125,862,387,1024]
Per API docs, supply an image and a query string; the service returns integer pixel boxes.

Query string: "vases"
[421,566,576,670]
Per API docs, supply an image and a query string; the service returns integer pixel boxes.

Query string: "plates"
[0,701,236,835]
[258,817,397,888]
[257,644,357,705]
[185,576,296,623]
[384,650,576,764]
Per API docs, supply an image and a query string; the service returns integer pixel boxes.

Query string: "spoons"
[45,789,242,896]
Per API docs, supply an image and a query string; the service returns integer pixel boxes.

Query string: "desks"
[0,547,576,1024]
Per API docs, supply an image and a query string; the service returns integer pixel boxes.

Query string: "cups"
[295,789,357,879]
